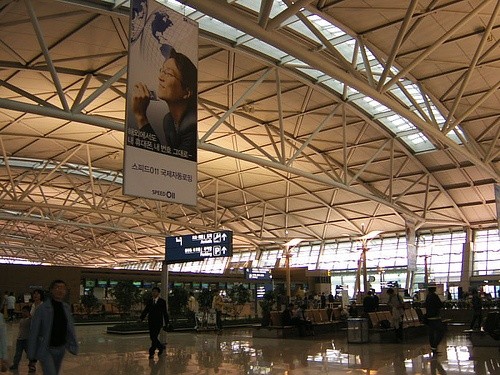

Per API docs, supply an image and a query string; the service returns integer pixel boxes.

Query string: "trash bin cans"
[346,318,369,344]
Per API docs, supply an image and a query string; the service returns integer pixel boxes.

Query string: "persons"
[131,43,197,162]
[0,279,500,375]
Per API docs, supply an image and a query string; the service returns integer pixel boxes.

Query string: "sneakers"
[28,364,36,372]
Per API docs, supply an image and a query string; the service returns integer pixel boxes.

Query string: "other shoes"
[10,364,18,369]
[158,346,166,356]
[149,353,155,359]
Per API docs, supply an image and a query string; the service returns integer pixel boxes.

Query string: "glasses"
[159,68,182,81]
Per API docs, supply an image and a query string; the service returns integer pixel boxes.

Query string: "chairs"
[251,308,500,347]
